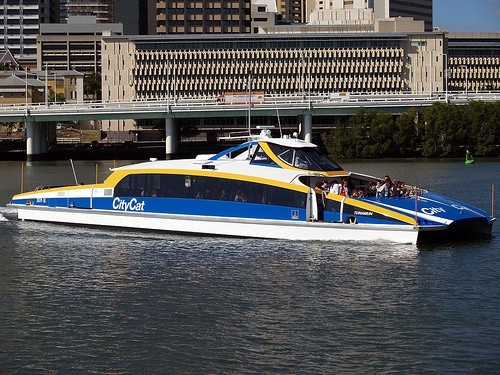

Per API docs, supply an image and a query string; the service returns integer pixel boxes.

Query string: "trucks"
[322,92,350,103]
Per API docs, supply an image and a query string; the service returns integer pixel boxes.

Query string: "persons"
[466,148,471,157]
[315,175,409,198]
[124,186,272,205]
[3,61,20,71]
[78,182,84,186]
[7,124,25,132]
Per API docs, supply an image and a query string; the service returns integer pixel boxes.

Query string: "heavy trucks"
[214,89,264,106]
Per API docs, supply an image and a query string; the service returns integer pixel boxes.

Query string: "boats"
[7,134,495,242]
[465,149,475,164]
